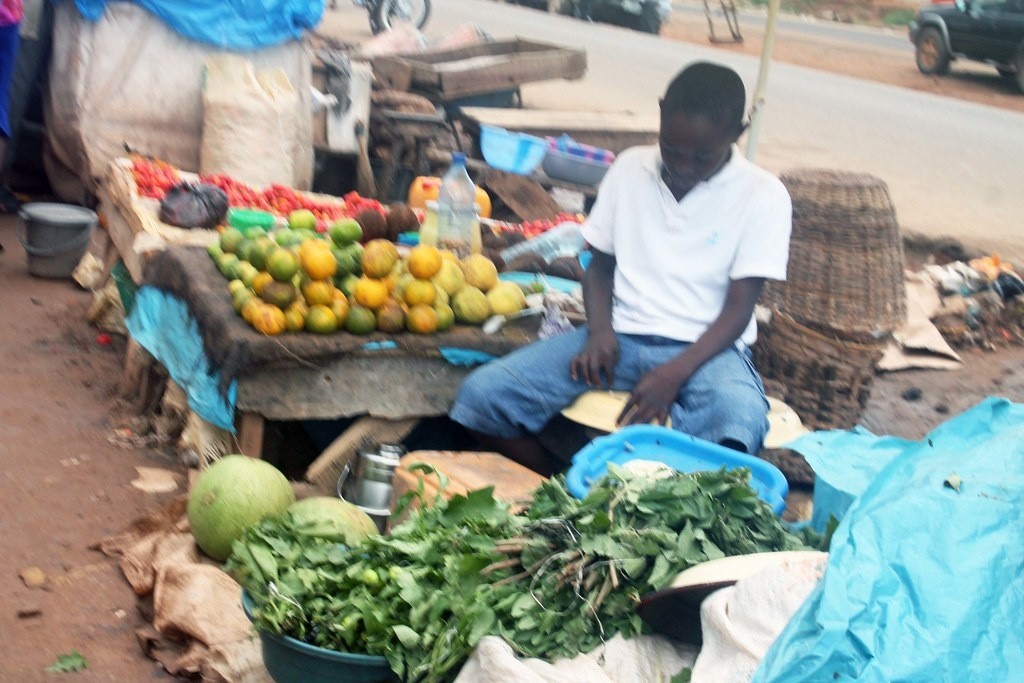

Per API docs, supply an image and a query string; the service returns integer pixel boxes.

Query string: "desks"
[85,163,540,463]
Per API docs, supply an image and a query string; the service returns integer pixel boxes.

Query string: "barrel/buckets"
[19,200,98,277]
[338,442,411,537]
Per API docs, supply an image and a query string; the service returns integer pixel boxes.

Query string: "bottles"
[437,152,476,259]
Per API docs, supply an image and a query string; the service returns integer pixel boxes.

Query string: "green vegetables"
[228,460,840,683]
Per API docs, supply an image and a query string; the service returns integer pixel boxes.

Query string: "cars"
[908,0,1024,93]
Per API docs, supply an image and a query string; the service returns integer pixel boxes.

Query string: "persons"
[449,61,793,479]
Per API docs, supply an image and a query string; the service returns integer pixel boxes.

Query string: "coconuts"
[188,455,380,561]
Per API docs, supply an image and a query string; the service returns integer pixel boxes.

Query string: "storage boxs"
[386,37,582,99]
[387,448,547,536]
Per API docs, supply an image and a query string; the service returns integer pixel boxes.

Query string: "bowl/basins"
[542,147,612,185]
[239,586,412,683]
[478,123,549,174]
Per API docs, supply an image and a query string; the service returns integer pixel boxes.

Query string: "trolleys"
[290,16,586,140]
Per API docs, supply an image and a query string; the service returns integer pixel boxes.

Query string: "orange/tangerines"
[208,209,525,335]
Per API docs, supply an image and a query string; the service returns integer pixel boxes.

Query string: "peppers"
[501,212,587,237]
[129,155,387,231]
